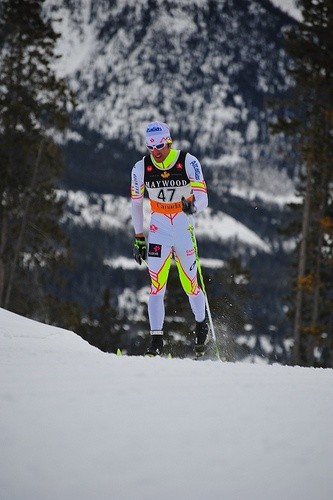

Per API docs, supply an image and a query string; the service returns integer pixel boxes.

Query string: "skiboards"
[118,348,220,359]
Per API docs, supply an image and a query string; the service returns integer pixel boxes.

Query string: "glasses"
[145,142,166,151]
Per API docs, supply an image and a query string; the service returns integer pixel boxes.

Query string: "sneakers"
[192,317,210,356]
[145,330,163,356]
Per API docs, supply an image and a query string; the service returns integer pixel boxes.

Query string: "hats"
[144,122,169,145]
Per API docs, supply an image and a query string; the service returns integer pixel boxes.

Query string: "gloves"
[132,236,146,266]
[180,196,194,215]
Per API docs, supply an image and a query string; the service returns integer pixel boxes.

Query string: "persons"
[129,120,209,362]
